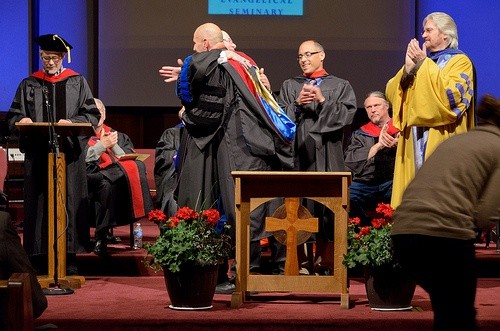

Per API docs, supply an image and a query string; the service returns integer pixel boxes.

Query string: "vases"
[362,262,415,306]
[162,262,218,309]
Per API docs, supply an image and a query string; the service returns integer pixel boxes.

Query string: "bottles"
[133,223,143,250]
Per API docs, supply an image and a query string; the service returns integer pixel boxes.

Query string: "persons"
[83,98,152,257]
[343,91,400,235]
[0,211,48,327]
[391,94,500,331]
[6,33,102,279]
[279,39,356,276]
[152,22,296,294]
[383,10,477,211]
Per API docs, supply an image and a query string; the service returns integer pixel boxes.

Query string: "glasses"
[41,54,60,62]
[297,50,322,61]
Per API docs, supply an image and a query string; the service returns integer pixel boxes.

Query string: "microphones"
[43,70,49,94]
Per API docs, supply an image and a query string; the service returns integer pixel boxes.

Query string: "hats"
[36,33,74,64]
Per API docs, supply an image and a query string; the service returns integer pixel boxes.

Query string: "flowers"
[140,178,236,274]
[340,202,401,270]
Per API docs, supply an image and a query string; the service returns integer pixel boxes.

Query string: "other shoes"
[270,260,330,276]
[213,280,236,295]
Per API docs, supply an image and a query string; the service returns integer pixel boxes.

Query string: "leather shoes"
[93,229,122,256]
